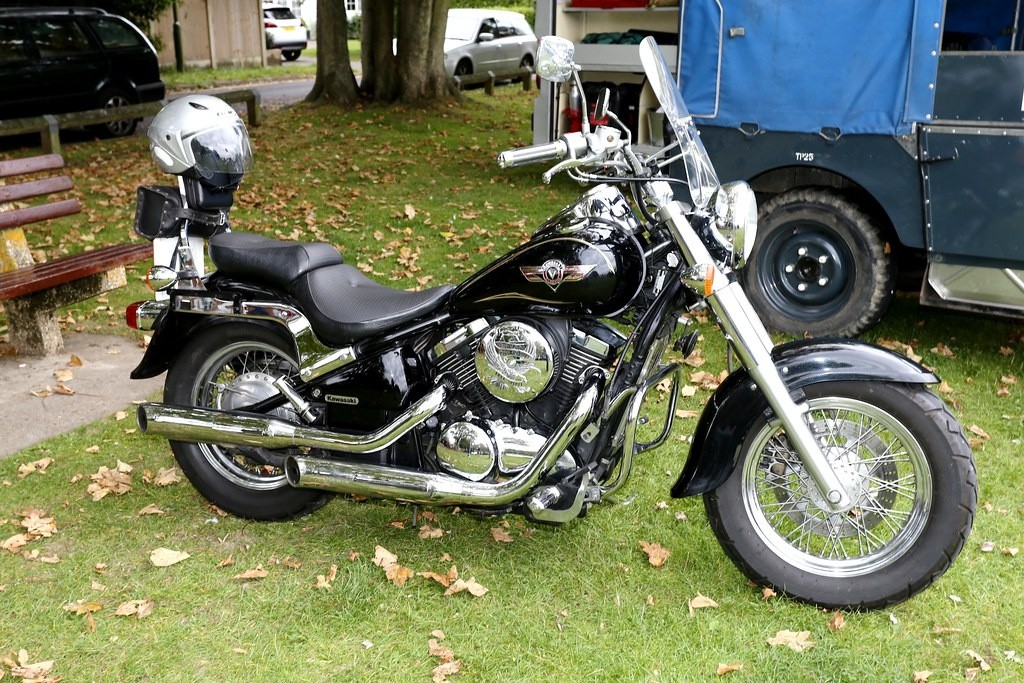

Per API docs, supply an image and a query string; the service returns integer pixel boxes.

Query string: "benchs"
[0,153,154,358]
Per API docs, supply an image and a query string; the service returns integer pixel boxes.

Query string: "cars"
[391,7,542,91]
[262,3,310,58]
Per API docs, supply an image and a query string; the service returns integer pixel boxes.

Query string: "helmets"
[147,95,254,190]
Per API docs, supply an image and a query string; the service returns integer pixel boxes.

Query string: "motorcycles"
[658,1,1024,341]
[126,33,978,617]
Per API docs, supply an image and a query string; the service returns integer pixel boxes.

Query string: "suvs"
[0,6,168,140]
